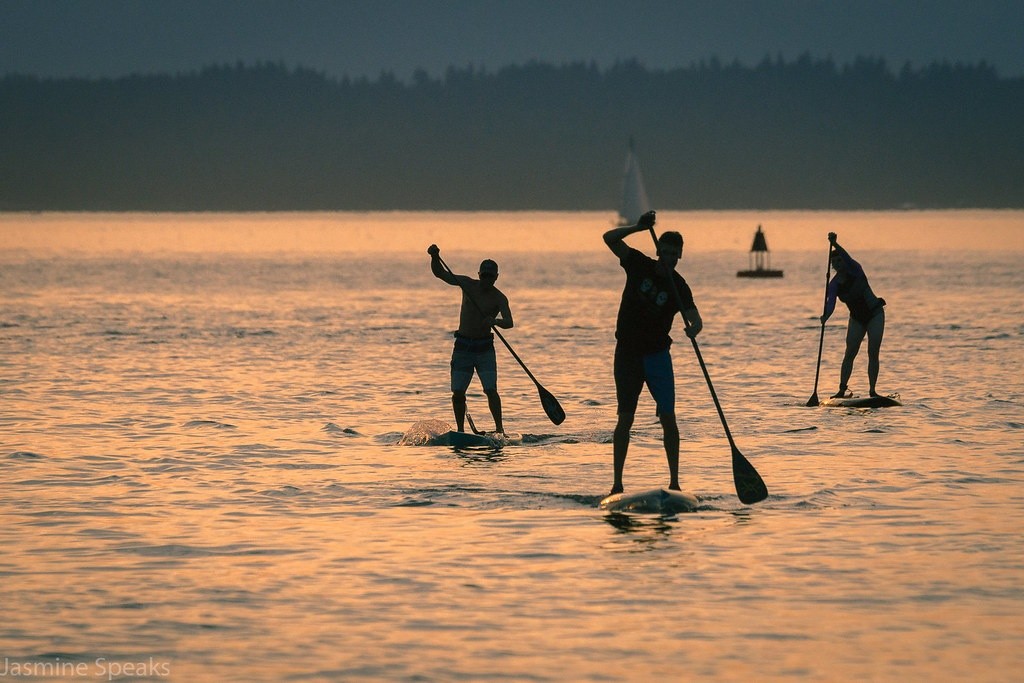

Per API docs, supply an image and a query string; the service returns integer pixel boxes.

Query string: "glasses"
[832,258,842,263]
[662,248,678,257]
[479,272,495,278]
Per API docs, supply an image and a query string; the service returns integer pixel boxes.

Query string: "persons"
[601,210,704,495]
[428,243,515,437]
[819,231,887,399]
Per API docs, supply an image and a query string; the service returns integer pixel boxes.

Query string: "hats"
[831,250,842,258]
[479,259,498,275]
[657,231,683,247]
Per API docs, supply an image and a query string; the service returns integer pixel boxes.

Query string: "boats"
[430,430,523,446]
[819,396,902,408]
[599,489,698,514]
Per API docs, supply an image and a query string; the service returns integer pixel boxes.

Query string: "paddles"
[648,224,768,505]
[439,256,566,426]
[805,238,831,407]
